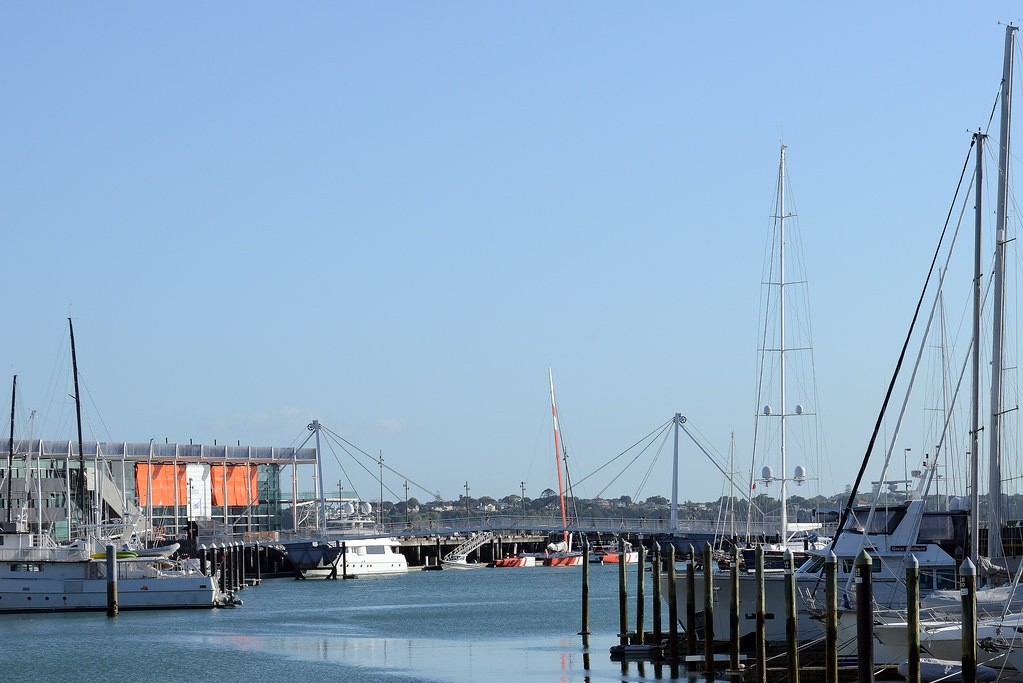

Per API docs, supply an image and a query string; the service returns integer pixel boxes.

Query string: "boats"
[416,413,832,574]
[298,537,409,581]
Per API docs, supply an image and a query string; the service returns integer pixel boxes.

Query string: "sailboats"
[0,316,221,609]
[618,20,1023,681]
